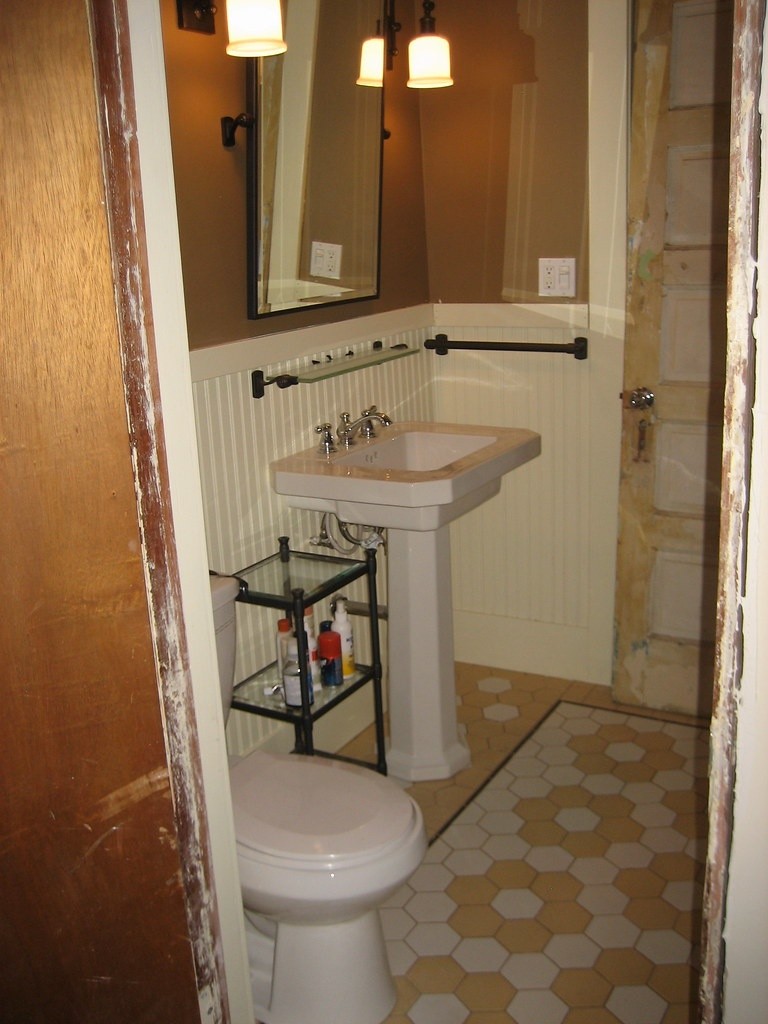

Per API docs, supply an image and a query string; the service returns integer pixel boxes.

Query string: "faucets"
[335,412,391,444]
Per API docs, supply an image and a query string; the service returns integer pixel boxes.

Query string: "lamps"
[407,0,454,89]
[176,0,288,57]
[356,19,384,87]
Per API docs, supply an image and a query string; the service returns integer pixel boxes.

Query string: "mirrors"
[221,0,401,320]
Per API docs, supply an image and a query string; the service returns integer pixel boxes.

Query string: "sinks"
[269,420,543,530]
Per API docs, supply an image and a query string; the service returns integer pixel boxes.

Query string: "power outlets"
[538,258,577,296]
[309,241,343,280]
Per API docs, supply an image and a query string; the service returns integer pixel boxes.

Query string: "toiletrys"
[275,598,355,708]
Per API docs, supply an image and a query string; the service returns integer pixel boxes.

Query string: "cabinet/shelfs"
[209,536,387,776]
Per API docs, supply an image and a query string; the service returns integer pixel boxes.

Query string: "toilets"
[208,577,422,1022]
